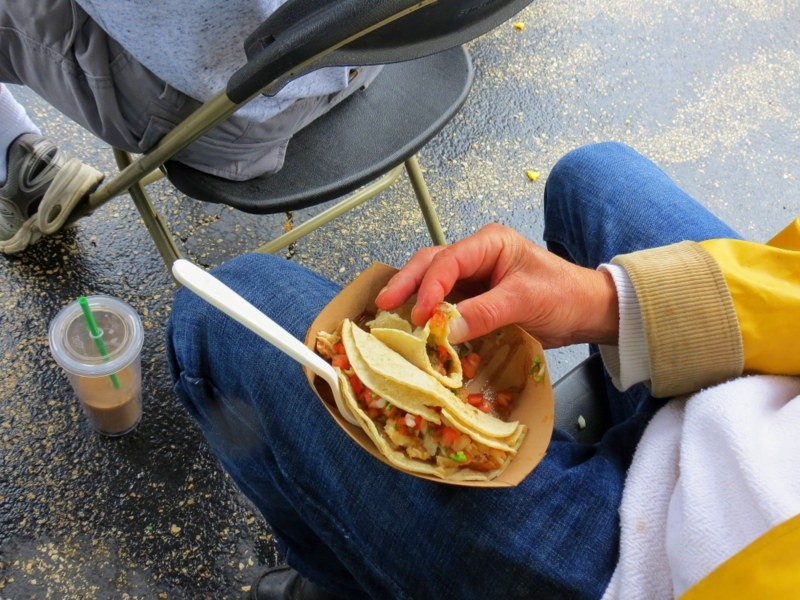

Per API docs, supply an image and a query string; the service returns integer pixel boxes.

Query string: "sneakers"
[0,132,104,255]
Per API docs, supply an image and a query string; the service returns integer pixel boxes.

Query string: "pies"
[334,301,527,480]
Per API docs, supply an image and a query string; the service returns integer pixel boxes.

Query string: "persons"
[166,140,800,600]
[1,1,386,255]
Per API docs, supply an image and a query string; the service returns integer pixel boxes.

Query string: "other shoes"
[250,565,333,600]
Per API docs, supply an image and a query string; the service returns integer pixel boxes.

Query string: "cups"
[48,295,145,437]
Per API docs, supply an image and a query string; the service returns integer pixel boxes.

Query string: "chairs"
[57,0,534,291]
